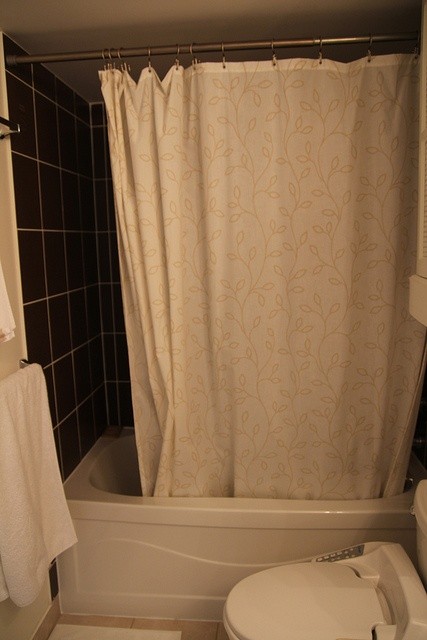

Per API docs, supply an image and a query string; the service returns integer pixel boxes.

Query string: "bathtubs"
[55,424,425,622]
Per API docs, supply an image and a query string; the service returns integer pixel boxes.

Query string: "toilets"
[224,477,427,640]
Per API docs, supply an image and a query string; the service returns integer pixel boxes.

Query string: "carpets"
[48,625,182,640]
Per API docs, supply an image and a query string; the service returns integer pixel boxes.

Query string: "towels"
[0,260,16,345]
[1,363,79,609]
